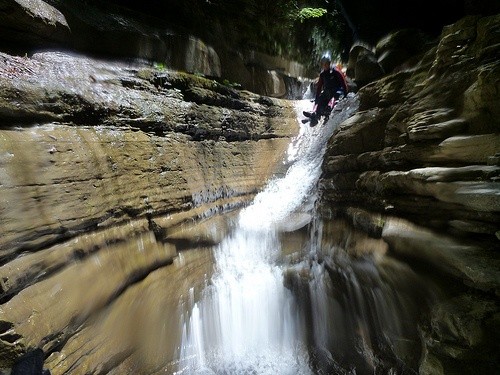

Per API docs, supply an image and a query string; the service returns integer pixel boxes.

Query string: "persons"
[298,54,349,127]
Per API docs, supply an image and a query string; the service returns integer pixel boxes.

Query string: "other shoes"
[321,109,330,117]
[303,111,317,120]
